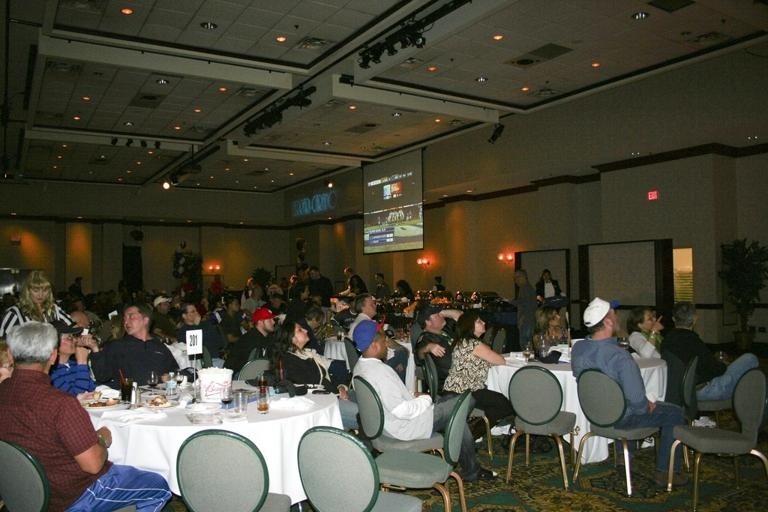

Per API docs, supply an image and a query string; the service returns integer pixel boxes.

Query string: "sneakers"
[694,416,716,428]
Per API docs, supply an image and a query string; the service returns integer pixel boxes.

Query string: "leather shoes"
[616,457,631,477]
[655,472,688,488]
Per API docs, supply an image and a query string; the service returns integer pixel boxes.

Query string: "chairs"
[654,356,699,472]
[176,429,290,512]
[481,328,494,351]
[238,361,274,386]
[373,390,467,512]
[353,378,444,463]
[425,356,493,461]
[1,441,137,512]
[492,328,507,354]
[506,367,576,488]
[344,338,360,373]
[570,371,660,496]
[297,426,422,512]
[668,369,768,512]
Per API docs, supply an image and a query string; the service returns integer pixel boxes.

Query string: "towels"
[272,396,316,412]
[188,402,248,426]
[104,408,167,426]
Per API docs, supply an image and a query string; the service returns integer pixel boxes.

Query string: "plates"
[81,400,130,409]
[186,403,222,413]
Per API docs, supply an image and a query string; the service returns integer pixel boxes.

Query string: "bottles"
[167,372,176,396]
[131,382,141,403]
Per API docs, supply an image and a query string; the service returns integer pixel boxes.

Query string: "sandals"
[464,469,497,481]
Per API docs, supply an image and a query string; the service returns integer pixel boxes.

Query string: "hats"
[353,314,385,352]
[50,321,83,336]
[584,297,620,327]
[252,307,279,321]
[418,307,442,323]
[154,296,172,307]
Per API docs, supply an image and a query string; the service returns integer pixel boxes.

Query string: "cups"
[120,376,134,402]
[221,387,233,409]
[619,337,631,350]
[257,393,270,415]
[234,392,248,416]
[175,369,184,385]
[523,345,531,362]
[257,375,268,387]
[147,370,159,395]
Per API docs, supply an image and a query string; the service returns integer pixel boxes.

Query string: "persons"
[535,269,564,306]
[218,295,250,349]
[507,270,538,353]
[244,287,266,313]
[624,306,663,362]
[443,311,512,448]
[351,319,476,445]
[0,338,15,380]
[47,319,96,396]
[348,293,377,341]
[396,279,415,305]
[331,298,361,334]
[289,282,323,318]
[69,273,196,310]
[305,266,333,308]
[70,312,89,330]
[433,275,446,291]
[663,299,759,402]
[572,299,688,486]
[1,269,84,340]
[339,266,368,296]
[175,303,225,368]
[149,296,181,342]
[415,308,473,398]
[265,318,360,433]
[210,274,226,306]
[374,208,414,223]
[207,296,226,325]
[374,272,390,299]
[222,308,326,381]
[223,307,276,381]
[0,321,172,512]
[241,278,256,309]
[288,276,300,299]
[263,285,285,315]
[77,300,199,387]
[533,306,571,350]
